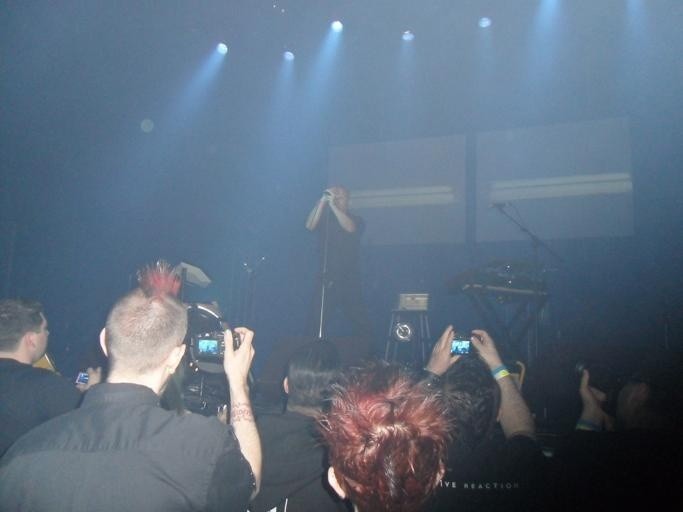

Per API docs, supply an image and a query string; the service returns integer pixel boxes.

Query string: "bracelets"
[576,418,592,424]
[494,370,511,380]
[576,424,593,431]
[491,367,504,375]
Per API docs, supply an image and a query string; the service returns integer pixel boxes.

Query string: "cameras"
[447,334,476,359]
[75,371,90,384]
[192,331,241,364]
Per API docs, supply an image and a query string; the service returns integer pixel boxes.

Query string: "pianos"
[451,260,566,300]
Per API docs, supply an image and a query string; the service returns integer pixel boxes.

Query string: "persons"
[248,340,351,510]
[304,185,374,344]
[2,258,262,511]
[314,324,557,512]
[3,297,102,454]
[576,353,681,511]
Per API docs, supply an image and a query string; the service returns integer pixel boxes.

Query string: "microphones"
[323,191,331,196]
[487,203,506,208]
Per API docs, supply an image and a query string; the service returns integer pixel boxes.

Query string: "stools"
[383,308,433,372]
